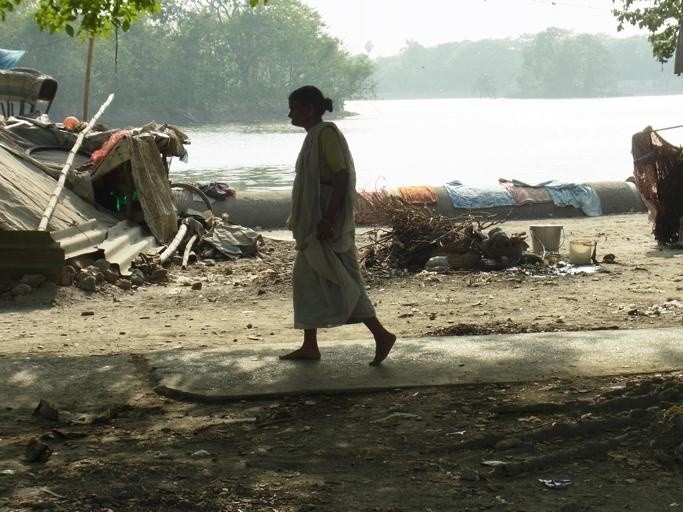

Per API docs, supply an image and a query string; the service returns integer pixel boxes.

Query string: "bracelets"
[319,217,335,233]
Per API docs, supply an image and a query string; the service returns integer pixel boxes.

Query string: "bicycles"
[166,141,213,221]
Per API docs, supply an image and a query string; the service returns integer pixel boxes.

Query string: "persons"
[276,86,397,366]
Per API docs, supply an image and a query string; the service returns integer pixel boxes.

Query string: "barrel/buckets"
[568,238,598,268]
[529,225,565,255]
